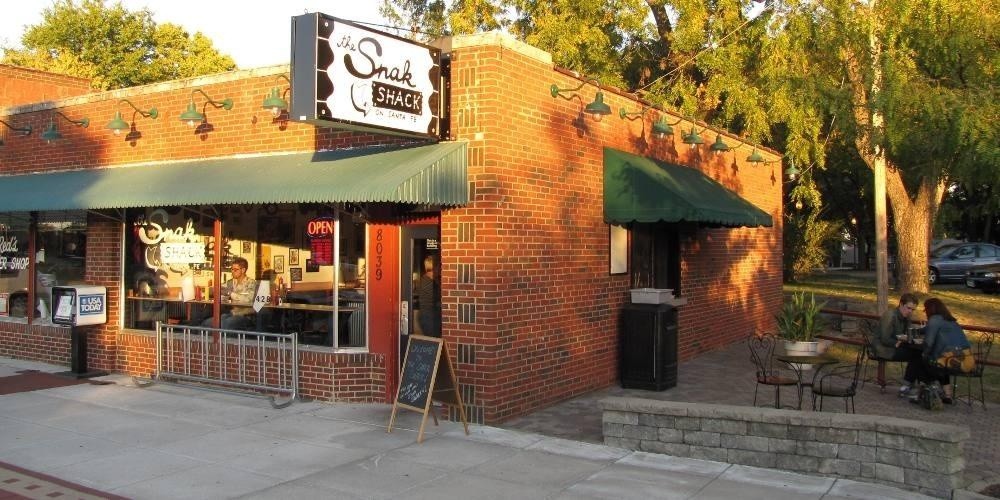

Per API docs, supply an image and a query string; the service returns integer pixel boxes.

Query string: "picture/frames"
[288,247,299,266]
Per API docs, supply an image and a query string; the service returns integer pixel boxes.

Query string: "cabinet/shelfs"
[619,305,679,390]
[273,255,284,274]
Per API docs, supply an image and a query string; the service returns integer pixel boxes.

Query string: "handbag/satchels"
[921,385,944,410]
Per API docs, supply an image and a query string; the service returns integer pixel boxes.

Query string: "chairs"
[809,336,872,413]
[745,331,795,410]
[856,318,905,393]
[944,330,994,413]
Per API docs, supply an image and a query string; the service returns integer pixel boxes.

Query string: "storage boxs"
[629,288,674,305]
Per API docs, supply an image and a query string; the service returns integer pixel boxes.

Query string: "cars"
[0,259,58,314]
[928,240,1000,286]
[891,239,967,277]
[962,261,1000,290]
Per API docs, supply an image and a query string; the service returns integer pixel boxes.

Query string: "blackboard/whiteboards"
[397,334,443,413]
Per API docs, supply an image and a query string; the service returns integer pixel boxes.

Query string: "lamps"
[728,136,764,168]
[551,74,613,124]
[0,117,32,148]
[104,97,159,137]
[179,87,233,129]
[38,110,90,147]
[619,102,675,138]
[764,148,801,181]
[668,115,704,149]
[262,74,292,118]
[698,124,729,155]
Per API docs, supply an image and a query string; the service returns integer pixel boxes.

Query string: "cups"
[193,264,200,269]
[240,294,249,303]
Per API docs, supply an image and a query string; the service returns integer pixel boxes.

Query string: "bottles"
[907,328,915,344]
[273,277,288,305]
[196,284,213,301]
[179,292,184,300]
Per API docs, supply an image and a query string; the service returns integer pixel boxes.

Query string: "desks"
[775,355,840,413]
[125,294,359,348]
[895,337,929,398]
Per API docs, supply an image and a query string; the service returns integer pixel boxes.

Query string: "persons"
[871,293,923,399]
[909,298,976,404]
[412,255,441,338]
[200,258,257,337]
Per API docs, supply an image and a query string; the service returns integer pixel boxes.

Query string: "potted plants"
[772,286,826,371]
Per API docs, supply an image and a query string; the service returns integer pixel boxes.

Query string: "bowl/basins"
[913,339,924,345]
[221,295,231,301]
[896,335,907,340]
[355,287,366,295]
